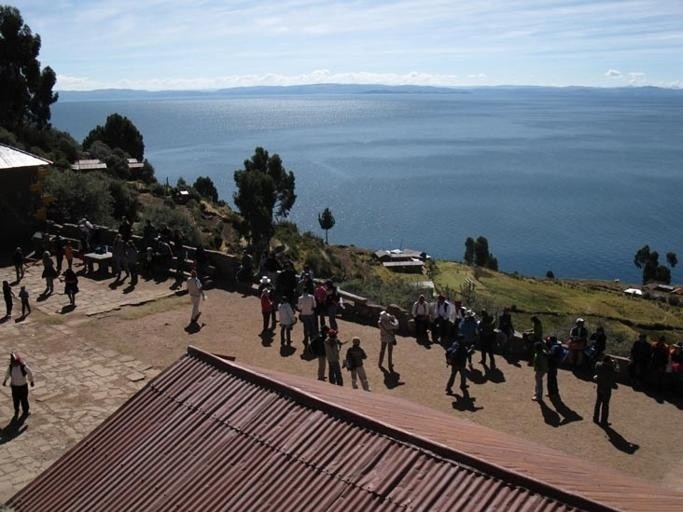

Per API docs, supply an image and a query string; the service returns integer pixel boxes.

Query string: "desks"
[83,252,113,276]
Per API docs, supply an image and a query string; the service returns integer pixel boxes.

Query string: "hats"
[259,276,271,284]
[319,325,329,334]
[326,328,338,337]
[464,310,475,318]
[378,310,392,321]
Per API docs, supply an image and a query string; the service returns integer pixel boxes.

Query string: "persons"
[0,213,206,316]
[376,303,401,369]
[1,352,35,418]
[186,269,207,323]
[234,246,371,392]
[410,294,683,427]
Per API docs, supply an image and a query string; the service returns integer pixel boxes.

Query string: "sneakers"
[531,392,551,401]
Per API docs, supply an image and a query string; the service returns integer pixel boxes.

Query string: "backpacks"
[443,345,462,368]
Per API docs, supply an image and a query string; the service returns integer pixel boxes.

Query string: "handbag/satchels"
[392,338,398,346]
